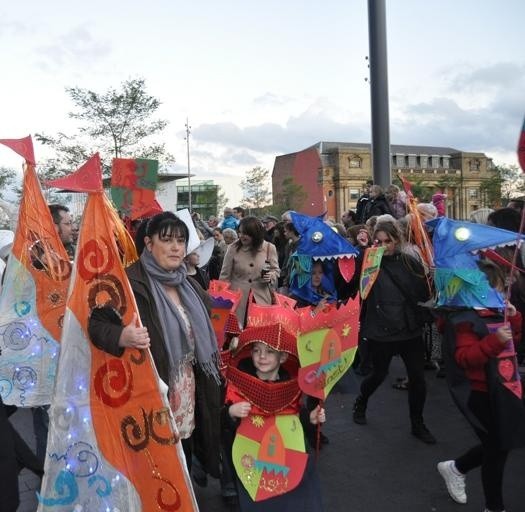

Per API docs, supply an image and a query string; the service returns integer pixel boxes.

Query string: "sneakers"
[222,487,237,505]
[192,462,207,488]
[392,376,426,390]
[352,403,367,425]
[437,368,448,378]
[424,362,438,370]
[437,461,467,505]
[411,425,436,444]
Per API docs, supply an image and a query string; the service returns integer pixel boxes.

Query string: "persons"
[435,259,525,511]
[351,215,439,445]
[31,205,78,458]
[135,182,523,391]
[88,212,221,479]
[222,342,326,512]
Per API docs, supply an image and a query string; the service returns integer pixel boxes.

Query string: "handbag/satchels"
[415,296,435,323]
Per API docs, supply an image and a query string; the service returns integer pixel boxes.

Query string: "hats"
[263,215,278,222]
